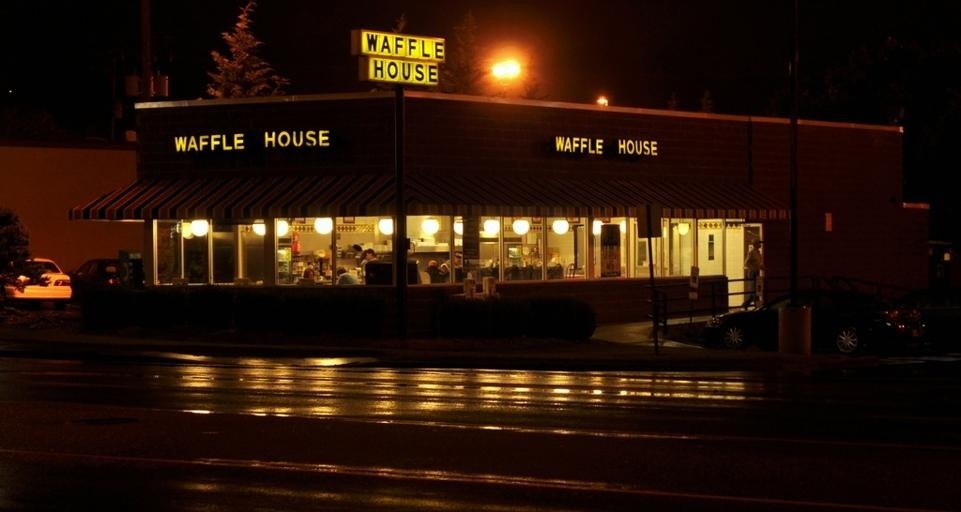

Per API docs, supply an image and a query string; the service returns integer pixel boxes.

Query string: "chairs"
[566,263,576,279]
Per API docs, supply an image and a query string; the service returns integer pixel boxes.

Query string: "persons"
[740,237,769,309]
[366,249,378,264]
[426,260,446,283]
[352,244,363,264]
[297,267,315,285]
[359,251,369,277]
[336,267,360,285]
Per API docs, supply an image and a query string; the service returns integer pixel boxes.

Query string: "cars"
[706,288,922,357]
[4,258,136,299]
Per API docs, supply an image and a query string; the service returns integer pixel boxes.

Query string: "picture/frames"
[293,218,306,223]
[343,217,355,223]
